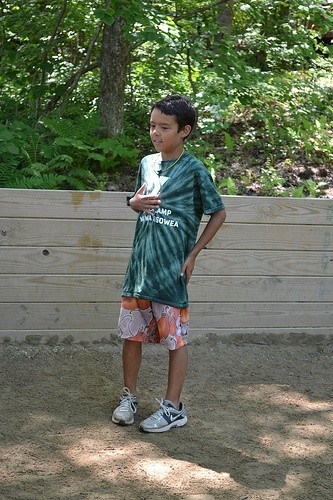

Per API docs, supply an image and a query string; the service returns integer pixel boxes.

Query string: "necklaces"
[157,148,184,174]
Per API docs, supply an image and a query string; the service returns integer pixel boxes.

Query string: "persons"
[111,94,227,433]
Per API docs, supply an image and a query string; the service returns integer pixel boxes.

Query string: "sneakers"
[112,387,137,425]
[140,397,188,432]
